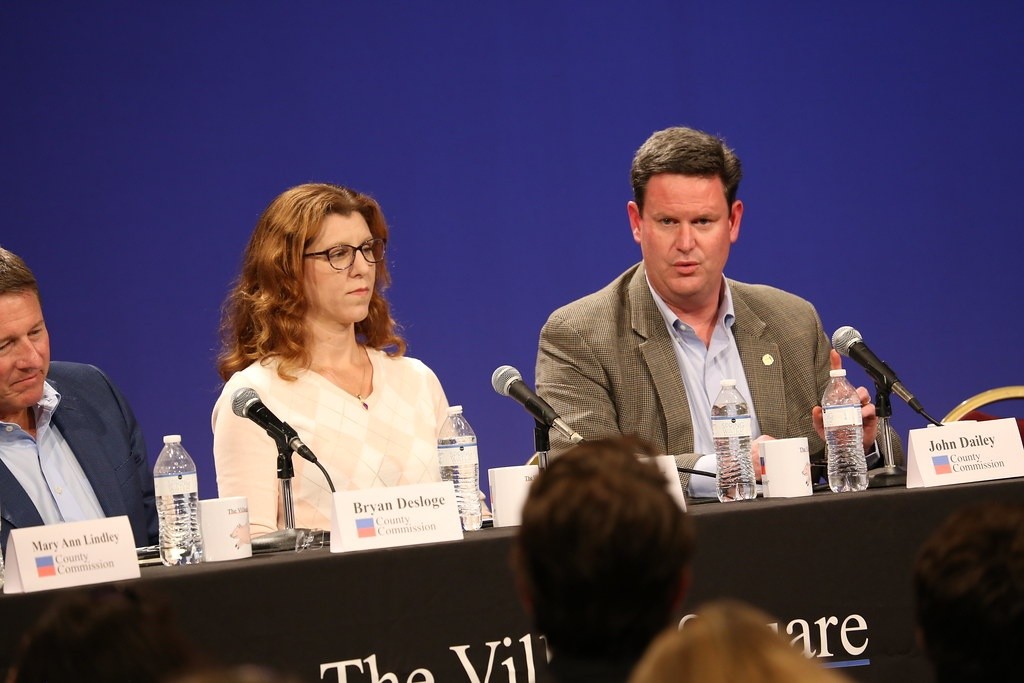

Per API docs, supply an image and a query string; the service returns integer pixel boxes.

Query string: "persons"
[211,184,493,538]
[0,248,159,564]
[519,438,848,683]
[536,127,902,498]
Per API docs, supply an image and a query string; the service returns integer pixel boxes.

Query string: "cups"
[758,437,813,497]
[196,496,252,562]
[488,465,539,527]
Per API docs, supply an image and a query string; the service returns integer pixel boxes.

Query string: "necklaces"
[307,345,366,401]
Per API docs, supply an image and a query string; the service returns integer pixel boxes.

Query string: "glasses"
[304,238,386,270]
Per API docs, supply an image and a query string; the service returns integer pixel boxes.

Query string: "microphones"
[832,326,924,414]
[230,387,317,463]
[491,365,587,445]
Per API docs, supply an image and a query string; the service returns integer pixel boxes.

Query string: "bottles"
[437,405,483,531]
[711,380,757,502]
[153,435,203,565]
[822,369,869,493]
